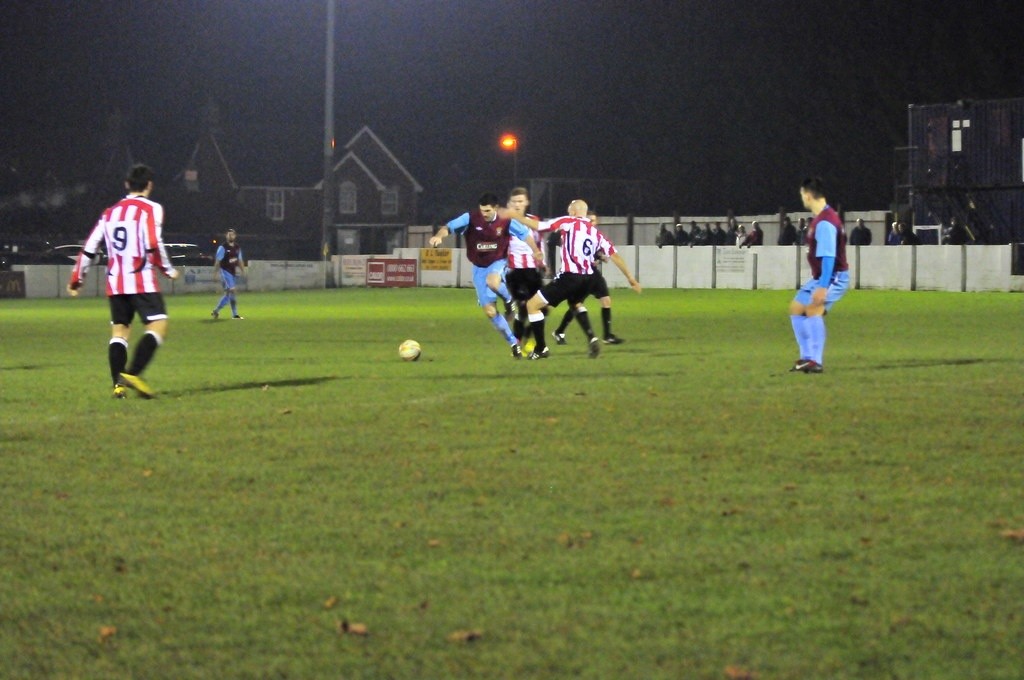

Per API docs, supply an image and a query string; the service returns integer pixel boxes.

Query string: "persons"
[942,217,966,245]
[67,164,178,400]
[654,217,763,249]
[502,185,539,354]
[212,229,246,320]
[789,176,850,371]
[850,218,872,245]
[508,200,641,358]
[428,193,543,358]
[887,221,921,245]
[552,209,626,344]
[777,216,813,245]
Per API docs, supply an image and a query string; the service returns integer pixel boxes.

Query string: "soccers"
[399,339,420,362]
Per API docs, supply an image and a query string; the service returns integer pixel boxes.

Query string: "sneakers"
[118,371,156,399]
[527,350,548,360]
[232,315,244,320]
[533,346,549,354]
[587,338,601,358]
[211,310,220,320]
[111,383,127,399]
[792,359,823,373]
[602,333,624,344]
[511,343,522,360]
[788,364,805,372]
[551,331,568,345]
